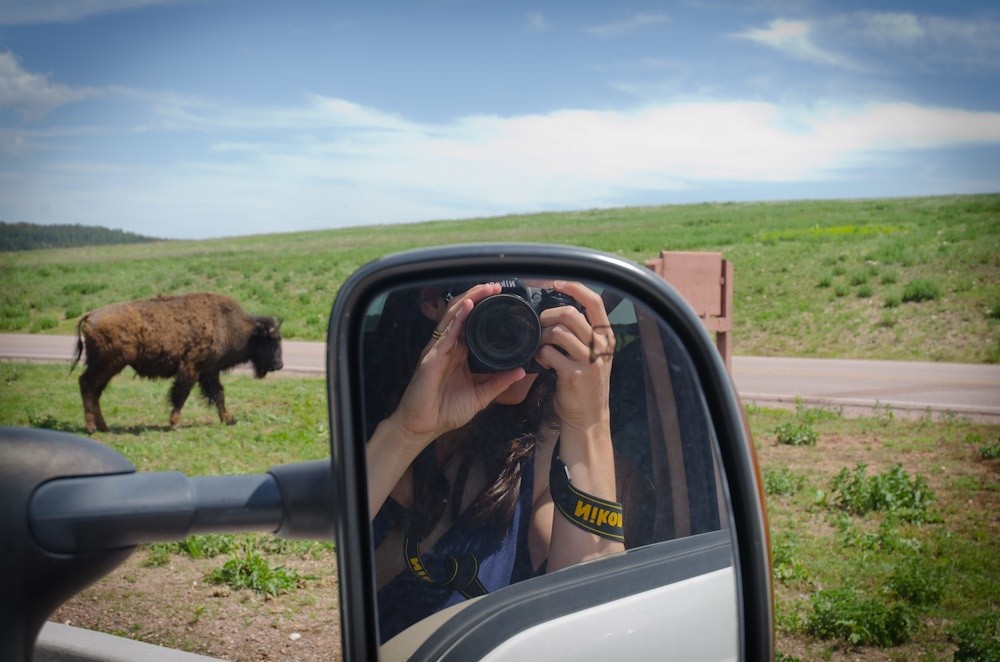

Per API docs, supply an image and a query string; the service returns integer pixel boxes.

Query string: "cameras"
[463,281,580,374]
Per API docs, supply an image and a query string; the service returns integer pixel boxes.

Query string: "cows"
[68,290,285,435]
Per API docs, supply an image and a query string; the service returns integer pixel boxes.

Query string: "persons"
[363,285,630,626]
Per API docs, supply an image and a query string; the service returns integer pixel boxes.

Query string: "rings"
[432,329,446,342]
